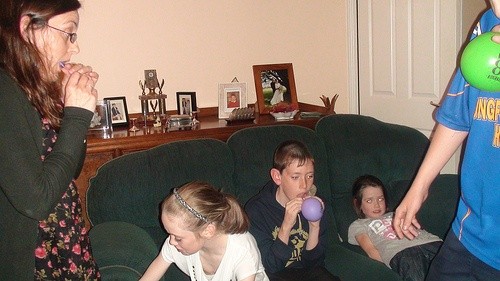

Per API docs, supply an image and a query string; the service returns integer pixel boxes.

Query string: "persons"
[270,75,288,105]
[393,0,500,281]
[111,102,124,120]
[244,139,405,281]
[348,173,445,281]
[0,0,98,281]
[182,98,190,114]
[136,177,269,281]
[226,94,240,108]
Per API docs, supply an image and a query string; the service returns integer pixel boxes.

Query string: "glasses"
[42,23,78,43]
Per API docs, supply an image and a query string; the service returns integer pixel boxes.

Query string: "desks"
[74,101,336,234]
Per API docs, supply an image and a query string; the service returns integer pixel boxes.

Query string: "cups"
[96,100,114,139]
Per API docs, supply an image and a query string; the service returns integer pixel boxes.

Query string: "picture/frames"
[103,95,131,129]
[176,92,199,121]
[252,62,298,114]
[218,81,248,119]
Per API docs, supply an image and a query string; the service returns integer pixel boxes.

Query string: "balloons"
[460,30,500,92]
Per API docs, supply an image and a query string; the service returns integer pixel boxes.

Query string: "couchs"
[86,113,462,281]
